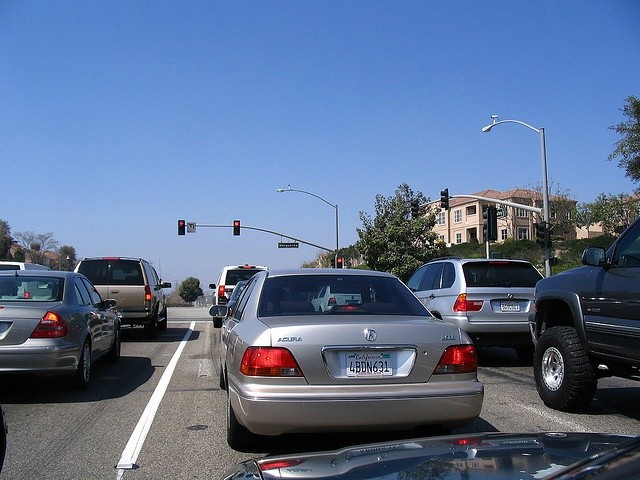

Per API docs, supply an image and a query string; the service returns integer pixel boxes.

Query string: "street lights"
[276,188,339,253]
[481,120,553,279]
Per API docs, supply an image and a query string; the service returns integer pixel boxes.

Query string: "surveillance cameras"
[491,115,498,118]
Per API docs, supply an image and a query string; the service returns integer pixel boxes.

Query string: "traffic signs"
[187,221,196,233]
[278,242,299,248]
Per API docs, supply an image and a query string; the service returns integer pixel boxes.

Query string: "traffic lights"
[534,221,553,248]
[440,187,449,211]
[233,219,241,236]
[177,219,186,235]
[330,255,344,268]
[482,206,498,240]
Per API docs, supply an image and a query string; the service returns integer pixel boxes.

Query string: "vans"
[209,264,268,328]
[0,261,53,299]
[73,256,172,338]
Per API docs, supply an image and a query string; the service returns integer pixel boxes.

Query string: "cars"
[217,280,250,324]
[216,431,638,479]
[209,267,485,452]
[0,270,122,384]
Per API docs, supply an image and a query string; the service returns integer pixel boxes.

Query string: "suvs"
[528,217,639,411]
[406,255,545,363]
